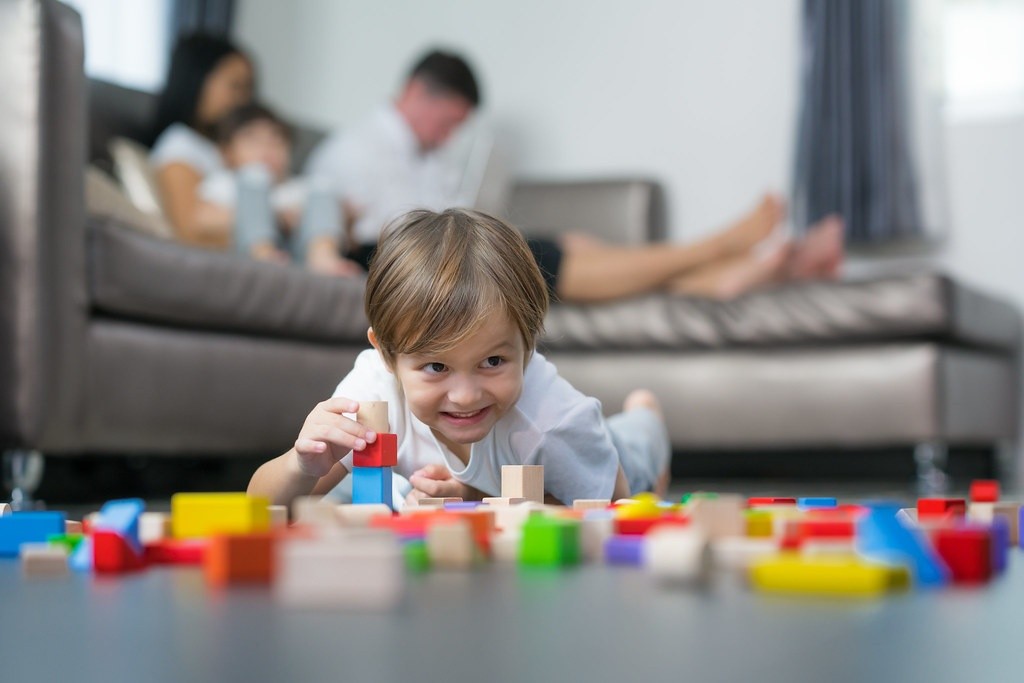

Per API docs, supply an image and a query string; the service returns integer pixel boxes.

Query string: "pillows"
[80,164,141,230]
[105,134,170,233]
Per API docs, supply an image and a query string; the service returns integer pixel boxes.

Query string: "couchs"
[0,0,1024,516]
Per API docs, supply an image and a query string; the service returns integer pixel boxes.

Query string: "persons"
[219,104,364,275]
[301,49,841,282]
[142,31,796,306]
[246,205,670,528]
